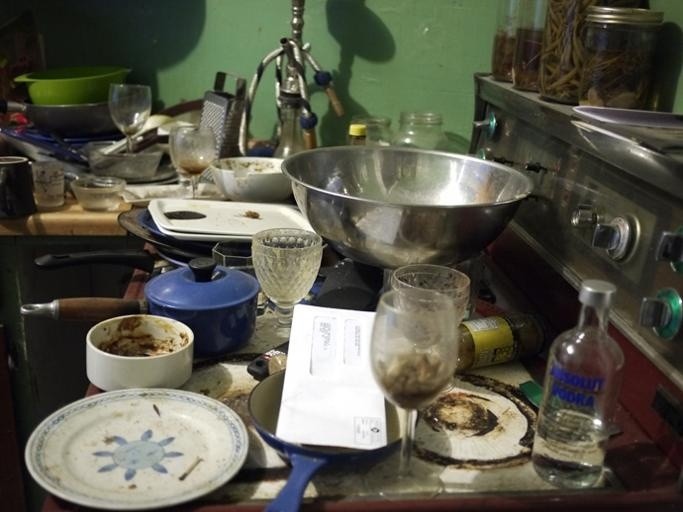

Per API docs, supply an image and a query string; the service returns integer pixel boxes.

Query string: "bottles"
[492,0,665,109]
[528,279,623,489]
[459,312,546,373]
[349,112,449,150]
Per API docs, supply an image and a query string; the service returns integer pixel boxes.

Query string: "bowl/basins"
[70,178,124,210]
[285,145,535,267]
[84,315,194,392]
[16,64,133,103]
[211,157,293,202]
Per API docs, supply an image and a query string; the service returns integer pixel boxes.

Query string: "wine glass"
[169,124,215,198]
[250,229,324,343]
[365,291,458,500]
[108,84,153,152]
[391,264,470,394]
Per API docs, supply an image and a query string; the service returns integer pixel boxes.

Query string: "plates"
[26,387,249,509]
[124,183,227,208]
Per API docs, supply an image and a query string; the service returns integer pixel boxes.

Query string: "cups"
[33,161,65,208]
[0,156,36,218]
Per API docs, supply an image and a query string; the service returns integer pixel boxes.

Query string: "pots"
[2,91,141,133]
[145,259,260,355]
[246,369,417,511]
